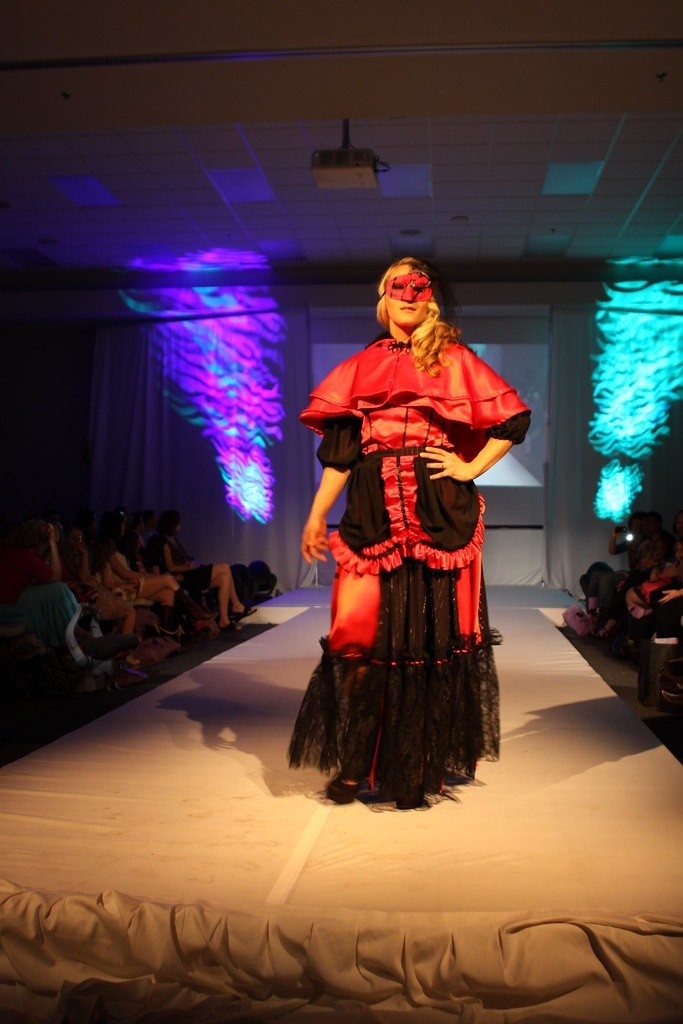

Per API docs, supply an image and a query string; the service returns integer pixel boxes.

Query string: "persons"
[147,509,257,632]
[0,506,220,695]
[298,258,532,812]
[563,508,683,717]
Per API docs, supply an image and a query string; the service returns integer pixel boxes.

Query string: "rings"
[441,463,445,469]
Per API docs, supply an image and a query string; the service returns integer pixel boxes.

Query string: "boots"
[161,587,220,626]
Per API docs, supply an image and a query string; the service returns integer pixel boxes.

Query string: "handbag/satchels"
[638,632,682,713]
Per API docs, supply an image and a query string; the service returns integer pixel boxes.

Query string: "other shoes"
[326,768,360,804]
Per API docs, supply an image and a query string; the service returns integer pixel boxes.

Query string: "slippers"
[218,608,256,633]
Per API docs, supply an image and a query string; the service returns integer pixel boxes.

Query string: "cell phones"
[614,526,627,533]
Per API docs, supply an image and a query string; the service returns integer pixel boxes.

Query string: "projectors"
[310,146,393,191]
